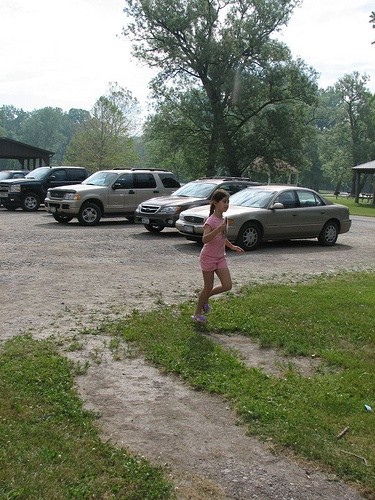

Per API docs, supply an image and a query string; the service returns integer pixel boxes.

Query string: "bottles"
[222,216,228,239]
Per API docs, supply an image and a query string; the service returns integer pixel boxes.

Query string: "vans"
[1,167,88,211]
[0,171,30,207]
[45,169,179,226]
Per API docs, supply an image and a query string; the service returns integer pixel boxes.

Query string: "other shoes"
[194,315,205,322]
[204,304,209,311]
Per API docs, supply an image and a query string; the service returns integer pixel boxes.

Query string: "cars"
[136,178,258,232]
[175,185,352,247]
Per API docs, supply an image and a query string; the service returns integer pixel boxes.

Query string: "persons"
[189,188,245,324]
